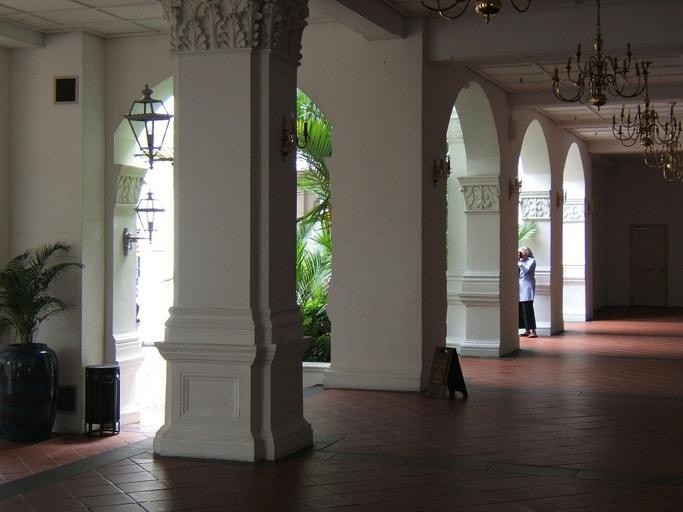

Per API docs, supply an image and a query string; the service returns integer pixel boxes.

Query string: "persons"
[517,246,538,338]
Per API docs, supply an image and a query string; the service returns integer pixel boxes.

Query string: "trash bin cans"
[85,364,120,437]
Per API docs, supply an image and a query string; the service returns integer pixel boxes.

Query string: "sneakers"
[520,333,528,336]
[528,335,537,338]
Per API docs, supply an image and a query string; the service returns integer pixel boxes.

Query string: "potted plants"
[0,240,86,444]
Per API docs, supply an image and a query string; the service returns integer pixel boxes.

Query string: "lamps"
[547,1,682,186]
[120,83,173,257]
